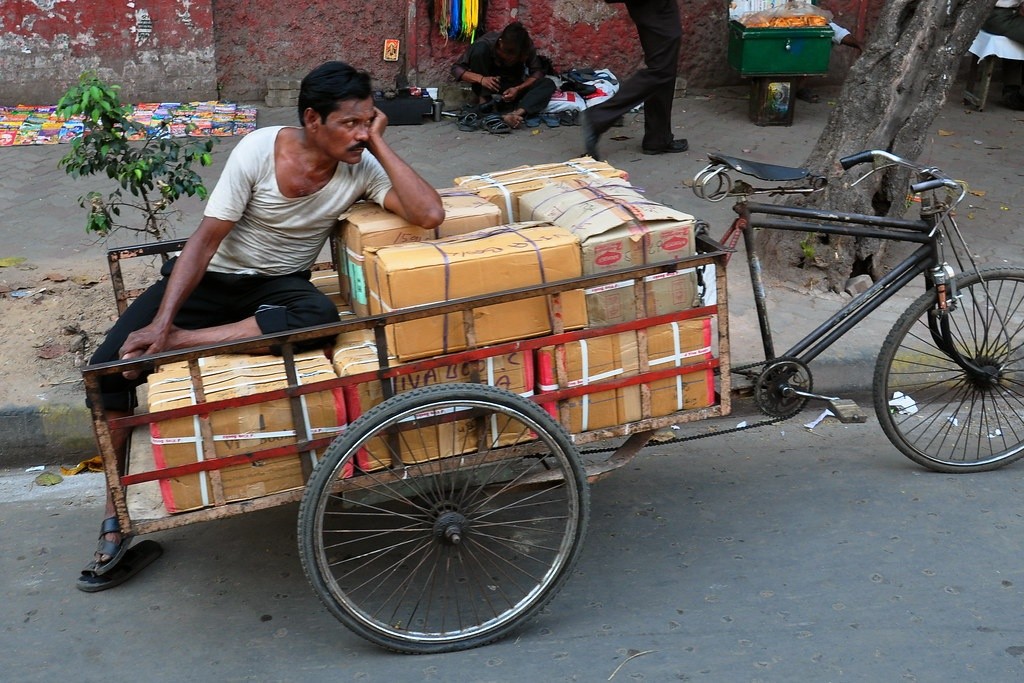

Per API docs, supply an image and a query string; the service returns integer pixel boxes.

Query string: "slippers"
[573,109,581,126]
[542,113,561,126]
[94,516,134,575]
[76,540,163,592]
[559,109,573,126]
[525,114,541,126]
[562,72,584,82]
[581,72,618,85]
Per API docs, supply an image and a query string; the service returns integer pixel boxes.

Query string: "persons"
[987,0,1024,111]
[581,0,689,158]
[82,61,446,578]
[794,21,865,102]
[448,23,558,129]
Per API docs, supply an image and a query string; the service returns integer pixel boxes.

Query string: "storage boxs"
[727,0,835,77]
[147,154,716,514]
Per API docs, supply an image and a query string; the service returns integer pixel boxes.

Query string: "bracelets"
[479,75,485,85]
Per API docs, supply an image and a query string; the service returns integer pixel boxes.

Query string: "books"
[0,101,259,145]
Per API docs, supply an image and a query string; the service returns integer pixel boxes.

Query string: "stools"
[962,28,1024,112]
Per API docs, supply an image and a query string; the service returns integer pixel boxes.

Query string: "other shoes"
[642,134,688,155]
[581,110,600,161]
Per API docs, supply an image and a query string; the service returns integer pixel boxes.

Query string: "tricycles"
[79,150,1024,656]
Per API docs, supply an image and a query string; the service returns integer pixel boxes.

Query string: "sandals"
[479,115,511,133]
[455,113,478,132]
